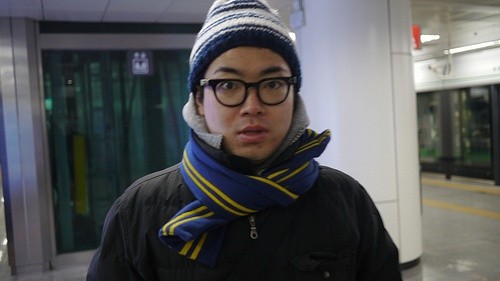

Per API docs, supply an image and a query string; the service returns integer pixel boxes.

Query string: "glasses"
[201,76,300,108]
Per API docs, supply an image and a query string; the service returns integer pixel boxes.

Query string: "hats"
[185,0,303,93]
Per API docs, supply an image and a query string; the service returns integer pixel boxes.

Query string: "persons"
[83,0,404,280]
